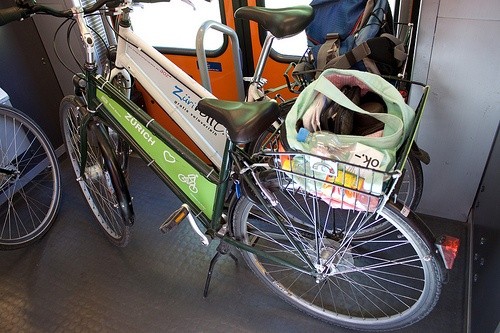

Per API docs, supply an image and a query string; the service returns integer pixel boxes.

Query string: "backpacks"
[292,0,408,91]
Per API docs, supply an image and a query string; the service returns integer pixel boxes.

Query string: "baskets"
[292,22,414,99]
[262,67,430,220]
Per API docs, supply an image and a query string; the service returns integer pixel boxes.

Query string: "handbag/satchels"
[285,68,415,213]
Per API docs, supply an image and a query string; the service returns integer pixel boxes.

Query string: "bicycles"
[1,102,64,252]
[103,0,432,242]
[1,1,461,333]
